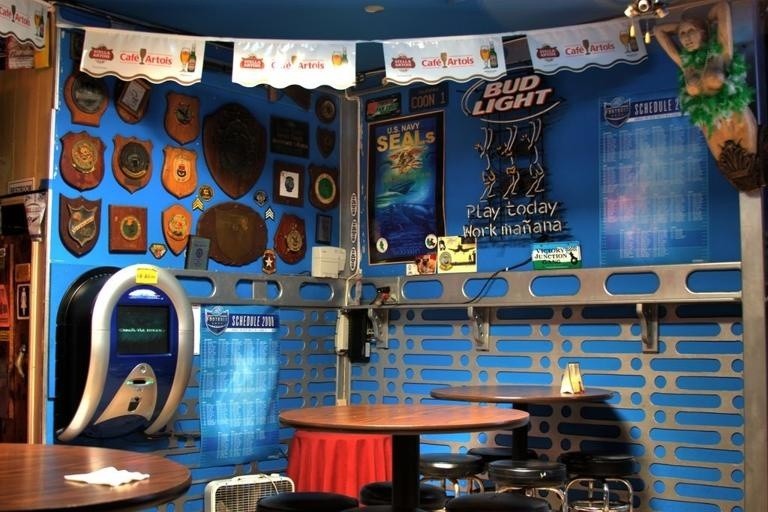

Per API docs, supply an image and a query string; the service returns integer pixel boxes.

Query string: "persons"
[650,0,768,191]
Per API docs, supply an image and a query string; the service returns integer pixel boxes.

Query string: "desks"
[277,404,529,512]
[429,384,613,492]
[0,443,193,512]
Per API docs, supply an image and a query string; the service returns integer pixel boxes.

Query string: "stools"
[254,446,638,512]
